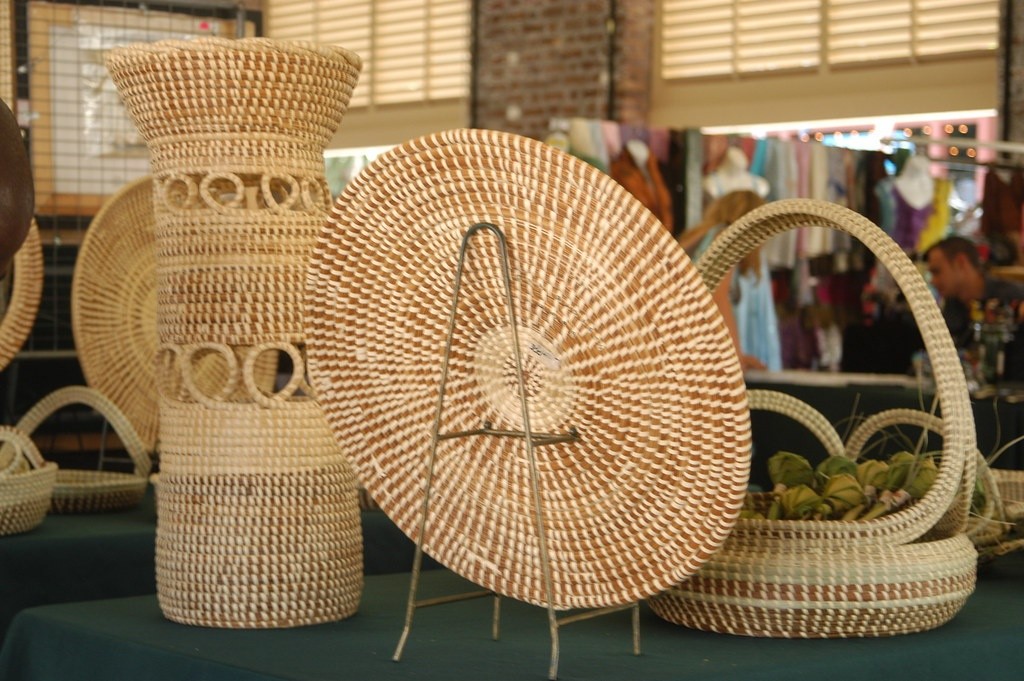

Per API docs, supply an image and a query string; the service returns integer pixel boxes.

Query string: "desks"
[746,368,1024,491]
[0,484,761,650]
[0,554,1024,681]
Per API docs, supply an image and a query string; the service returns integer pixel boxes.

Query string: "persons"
[675,190,770,375]
[922,234,1024,382]
[865,290,926,379]
[608,136,676,234]
[699,147,783,369]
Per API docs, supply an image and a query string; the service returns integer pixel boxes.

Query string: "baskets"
[981,436,1024,521]
[645,196,980,638]
[846,408,1024,558]
[7,386,151,512]
[0,426,59,535]
